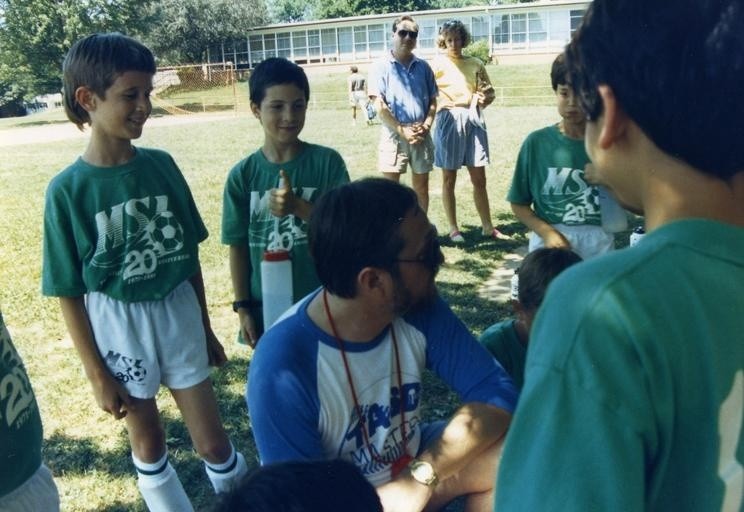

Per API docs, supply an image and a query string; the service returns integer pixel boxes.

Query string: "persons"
[426,20,513,243]
[479,248,583,392]
[221,58,352,349]
[209,460,384,512]
[349,66,370,126]
[0,312,62,512]
[42,32,249,512]
[366,15,439,216]
[506,50,617,264]
[248,176,517,512]
[494,0,744,512]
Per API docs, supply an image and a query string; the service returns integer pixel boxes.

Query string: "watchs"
[407,458,439,490]
[233,300,252,313]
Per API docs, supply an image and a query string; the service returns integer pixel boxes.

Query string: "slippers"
[449,231,465,242]
[482,229,509,240]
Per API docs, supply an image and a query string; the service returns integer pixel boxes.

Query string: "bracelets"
[426,124,431,129]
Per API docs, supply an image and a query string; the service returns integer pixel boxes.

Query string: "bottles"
[262,250,295,330]
[599,185,628,234]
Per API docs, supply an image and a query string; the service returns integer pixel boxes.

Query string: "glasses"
[397,240,439,267]
[398,30,417,37]
